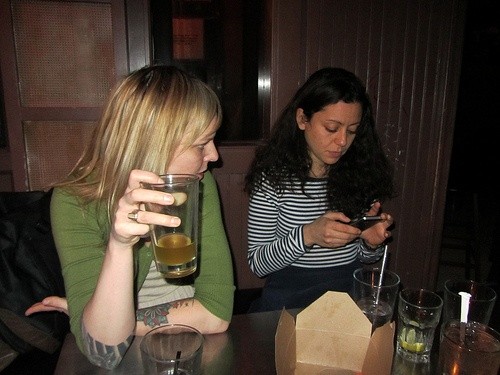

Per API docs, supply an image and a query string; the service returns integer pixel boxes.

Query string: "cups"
[438,321,500,375]
[138,173,199,277]
[352,267,399,332]
[140,325,203,375]
[442,279,495,336]
[395,288,442,362]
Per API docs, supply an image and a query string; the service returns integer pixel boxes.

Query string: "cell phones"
[348,215,382,230]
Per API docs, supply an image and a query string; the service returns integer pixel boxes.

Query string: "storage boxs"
[274,292,395,375]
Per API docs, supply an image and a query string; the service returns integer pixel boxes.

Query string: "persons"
[242,68,393,311]
[26,58,236,370]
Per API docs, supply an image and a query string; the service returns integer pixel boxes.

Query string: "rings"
[128,209,142,222]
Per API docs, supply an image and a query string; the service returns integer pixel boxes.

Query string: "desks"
[54,295,500,375]
